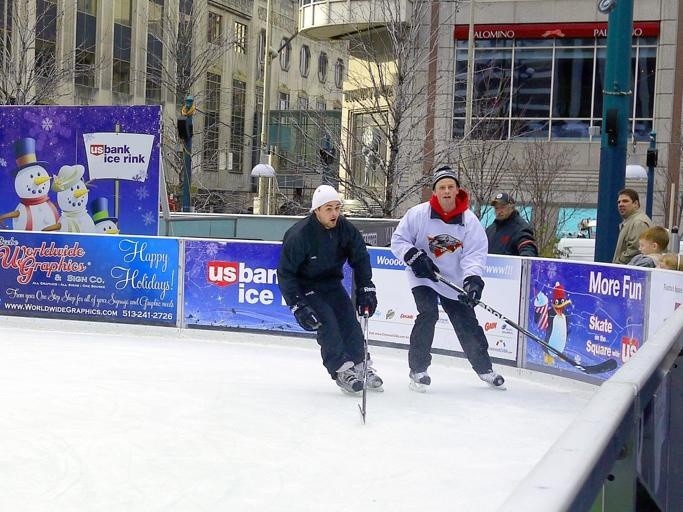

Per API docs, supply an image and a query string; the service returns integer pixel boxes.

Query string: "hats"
[309,184,341,213]
[490,193,514,206]
[432,165,459,190]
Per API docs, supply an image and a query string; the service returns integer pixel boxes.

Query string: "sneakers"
[478,371,503,386]
[352,360,382,388]
[408,369,430,385]
[334,361,362,392]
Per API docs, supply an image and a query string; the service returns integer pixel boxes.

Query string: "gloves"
[355,284,376,316]
[290,299,321,331]
[404,247,439,282]
[457,275,483,308]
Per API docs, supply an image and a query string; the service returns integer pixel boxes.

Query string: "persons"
[609,188,653,265]
[629,225,671,268]
[386,166,508,387]
[481,190,537,257]
[654,254,683,271]
[276,184,386,393]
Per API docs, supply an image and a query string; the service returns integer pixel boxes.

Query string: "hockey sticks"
[434,272,617,374]
[358,306,369,423]
